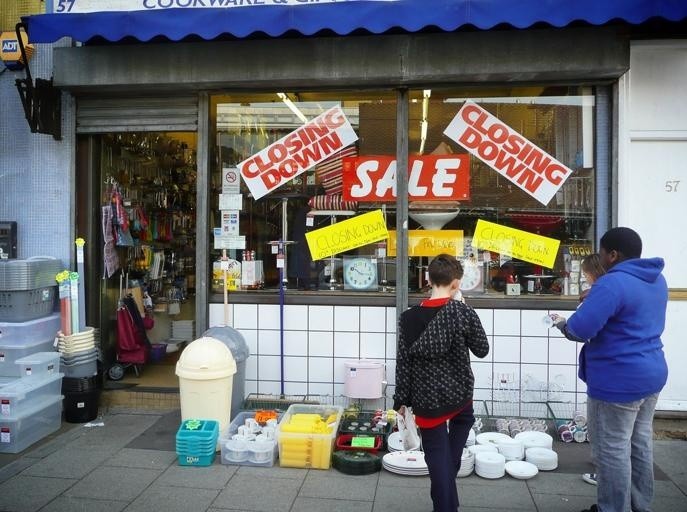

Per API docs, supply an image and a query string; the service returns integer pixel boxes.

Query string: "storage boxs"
[0,256,98,454]
[175,326,393,465]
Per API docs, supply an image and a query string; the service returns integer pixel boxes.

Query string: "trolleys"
[109,272,151,380]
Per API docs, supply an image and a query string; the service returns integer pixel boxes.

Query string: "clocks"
[342,254,378,291]
[455,263,485,293]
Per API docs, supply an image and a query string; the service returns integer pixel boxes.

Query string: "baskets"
[0,260,62,323]
[472,398,591,445]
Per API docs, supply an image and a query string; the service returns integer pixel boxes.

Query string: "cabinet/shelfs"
[102,132,195,339]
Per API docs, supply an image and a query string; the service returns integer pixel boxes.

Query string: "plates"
[380,428,559,480]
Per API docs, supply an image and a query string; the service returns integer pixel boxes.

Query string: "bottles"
[243,250,257,261]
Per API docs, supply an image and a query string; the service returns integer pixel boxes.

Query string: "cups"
[495,412,590,443]
[227,418,278,462]
[543,314,560,328]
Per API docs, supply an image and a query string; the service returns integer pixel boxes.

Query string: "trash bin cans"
[174,325,249,453]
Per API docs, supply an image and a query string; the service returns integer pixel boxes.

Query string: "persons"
[390,252,490,511]
[550,249,605,484]
[551,225,668,511]
